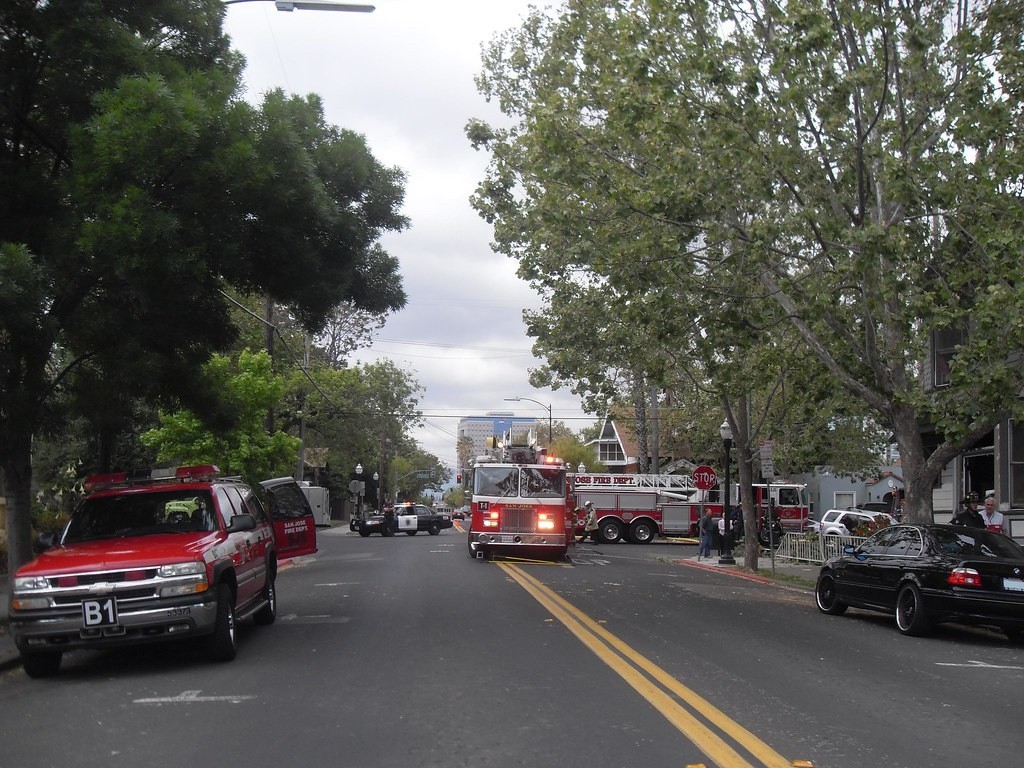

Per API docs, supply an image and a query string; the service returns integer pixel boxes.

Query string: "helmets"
[959,491,981,504]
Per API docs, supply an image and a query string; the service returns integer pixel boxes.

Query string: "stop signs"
[693,465,717,490]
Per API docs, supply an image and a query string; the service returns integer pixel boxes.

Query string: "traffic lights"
[456,474,462,483]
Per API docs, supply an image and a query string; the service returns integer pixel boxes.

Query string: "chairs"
[167,508,209,526]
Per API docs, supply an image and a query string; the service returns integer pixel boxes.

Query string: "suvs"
[7,464,318,677]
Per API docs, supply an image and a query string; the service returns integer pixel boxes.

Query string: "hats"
[585,501,591,506]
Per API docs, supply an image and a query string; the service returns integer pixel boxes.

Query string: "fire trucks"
[466,447,569,561]
[568,471,809,546]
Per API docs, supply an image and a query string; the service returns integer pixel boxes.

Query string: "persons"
[948,491,986,529]
[978,496,1007,535]
[381,497,395,537]
[576,500,600,545]
[697,502,744,558]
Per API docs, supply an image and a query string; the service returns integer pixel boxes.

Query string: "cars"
[350,502,454,537]
[819,508,900,558]
[452,510,465,521]
[815,521,1024,637]
[460,506,471,517]
[861,501,894,518]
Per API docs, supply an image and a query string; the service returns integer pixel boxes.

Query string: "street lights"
[717,417,738,566]
[372,471,379,512]
[503,396,553,447]
[355,463,364,520]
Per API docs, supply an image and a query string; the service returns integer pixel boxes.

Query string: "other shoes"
[718,552,720,556]
[592,542,598,545]
[705,555,713,558]
[576,540,582,543]
